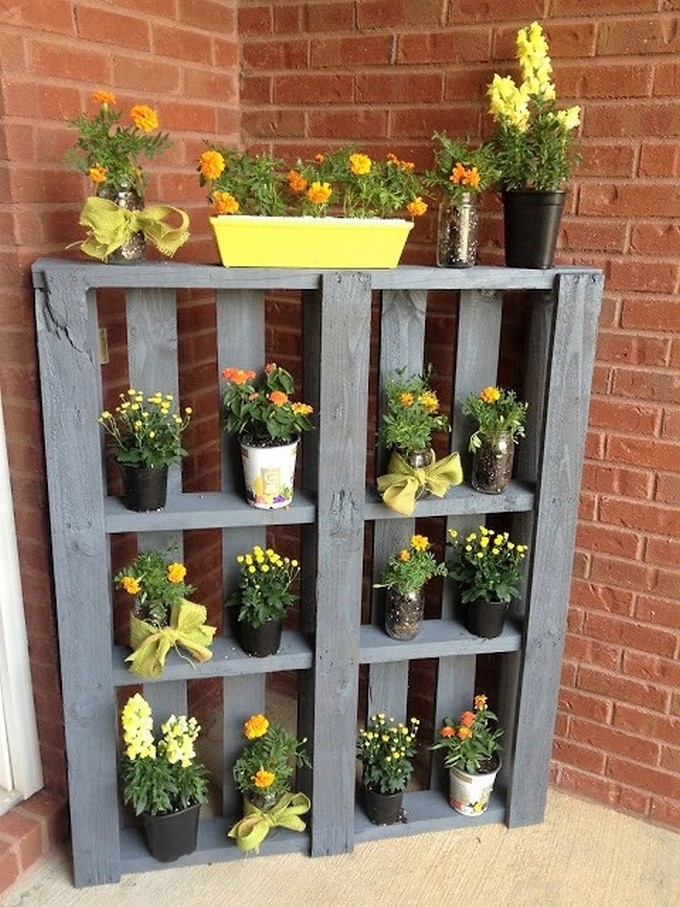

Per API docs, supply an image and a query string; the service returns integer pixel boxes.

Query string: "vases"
[241,436,300,510]
[467,591,507,638]
[97,168,146,263]
[119,464,168,512]
[435,191,479,268]
[364,786,403,825]
[385,589,424,641]
[130,597,171,659]
[450,752,504,817]
[242,790,285,839]
[140,795,201,863]
[240,618,282,657]
[387,446,436,501]
[471,430,514,494]
[501,191,569,270]
[209,214,414,269]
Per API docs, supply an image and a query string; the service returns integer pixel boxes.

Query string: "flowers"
[426,695,505,773]
[233,714,313,799]
[225,546,300,629]
[97,388,192,467]
[222,365,315,441]
[423,130,502,193]
[119,693,212,815]
[372,534,448,600]
[445,525,529,602]
[356,711,420,792]
[58,88,171,183]
[484,21,580,188]
[114,537,198,619]
[191,138,430,219]
[457,386,528,450]
[382,362,449,449]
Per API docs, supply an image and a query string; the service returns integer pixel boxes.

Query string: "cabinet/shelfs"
[29,258,605,889]
